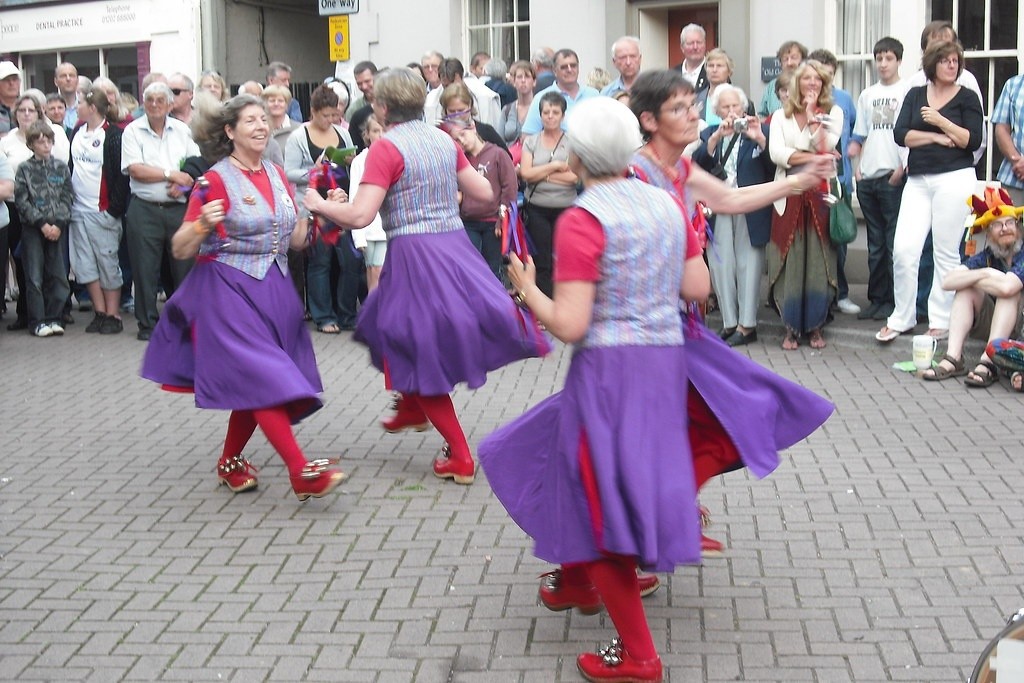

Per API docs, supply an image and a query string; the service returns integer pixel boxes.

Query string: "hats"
[0,61,19,80]
[965,186,1024,235]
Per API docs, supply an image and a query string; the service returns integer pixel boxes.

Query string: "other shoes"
[289,459,345,502]
[218,454,257,492]
[538,569,601,616]
[381,398,431,432]
[433,446,475,485]
[700,535,723,557]
[698,506,711,529]
[637,576,658,597]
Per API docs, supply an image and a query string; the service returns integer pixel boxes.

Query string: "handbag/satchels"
[816,177,857,246]
[518,206,530,227]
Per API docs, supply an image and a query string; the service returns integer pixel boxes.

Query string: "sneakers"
[0,282,166,340]
[713,298,951,350]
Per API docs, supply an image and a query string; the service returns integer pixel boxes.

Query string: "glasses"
[652,100,703,117]
[554,64,578,70]
[989,219,1019,232]
[170,88,189,97]
[936,57,959,64]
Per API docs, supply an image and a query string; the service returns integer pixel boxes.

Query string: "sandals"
[967,359,1001,388]
[316,321,339,334]
[923,354,968,379]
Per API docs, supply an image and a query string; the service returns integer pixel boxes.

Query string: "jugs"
[911,335,937,369]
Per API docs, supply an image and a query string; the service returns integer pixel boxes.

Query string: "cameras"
[733,118,748,132]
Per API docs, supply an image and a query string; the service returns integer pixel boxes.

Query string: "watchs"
[164,169,170,181]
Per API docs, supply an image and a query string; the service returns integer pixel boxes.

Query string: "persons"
[303,66,552,484]
[476,98,705,683]
[138,94,348,502]
[629,67,834,556]
[0,19,1024,391]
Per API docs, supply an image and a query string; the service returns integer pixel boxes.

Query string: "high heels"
[576,639,662,683]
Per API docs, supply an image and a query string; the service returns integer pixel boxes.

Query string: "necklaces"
[229,154,253,175]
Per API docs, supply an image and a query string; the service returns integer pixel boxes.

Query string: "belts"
[158,202,183,209]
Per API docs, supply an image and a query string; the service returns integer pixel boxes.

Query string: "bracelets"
[807,116,820,125]
[787,175,803,195]
[946,122,953,134]
[547,175,550,183]
[514,284,534,304]
